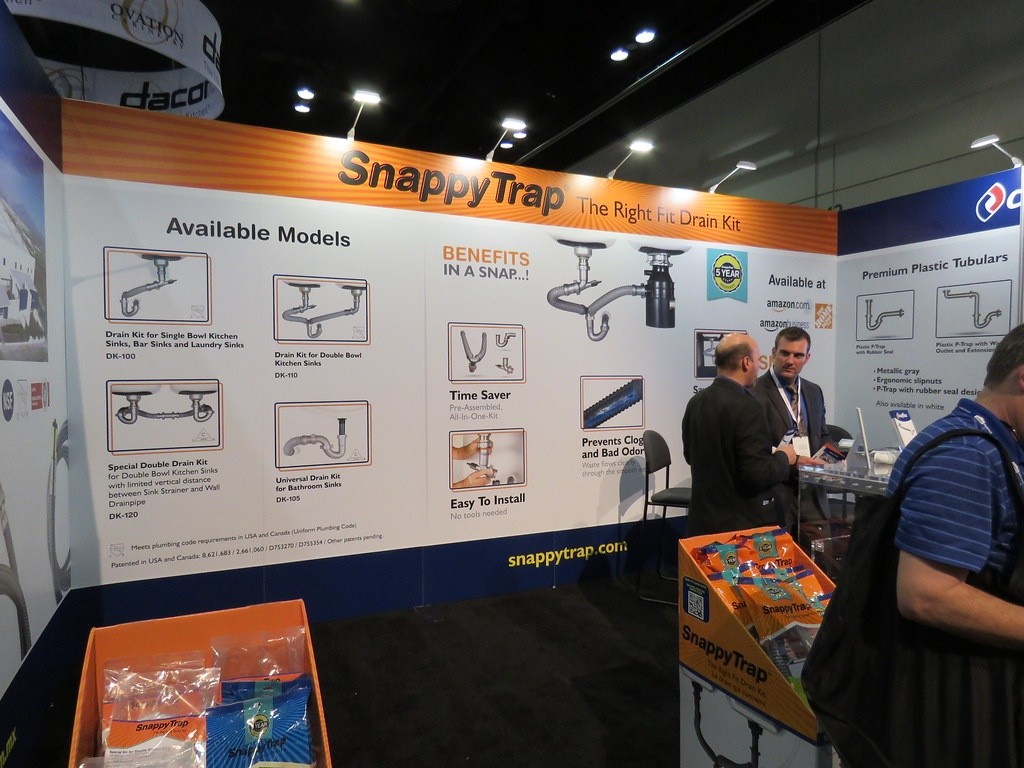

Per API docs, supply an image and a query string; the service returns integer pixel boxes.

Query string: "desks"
[797,455,898,548]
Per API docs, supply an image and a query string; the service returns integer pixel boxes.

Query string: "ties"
[787,385,804,437]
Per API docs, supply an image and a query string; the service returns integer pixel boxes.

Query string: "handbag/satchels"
[799,431,1024,768]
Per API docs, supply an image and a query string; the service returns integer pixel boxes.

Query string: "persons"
[682,334,797,537]
[839,323,1024,768]
[750,327,837,574]
[452,437,493,489]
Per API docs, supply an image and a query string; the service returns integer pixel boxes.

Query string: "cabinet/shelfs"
[68,598,332,768]
[677,524,842,768]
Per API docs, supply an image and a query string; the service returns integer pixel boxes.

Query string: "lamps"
[485,117,527,162]
[607,139,653,179]
[708,161,757,194]
[969,135,1023,166]
[346,90,380,140]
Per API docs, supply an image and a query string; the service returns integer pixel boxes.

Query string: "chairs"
[638,430,692,605]
